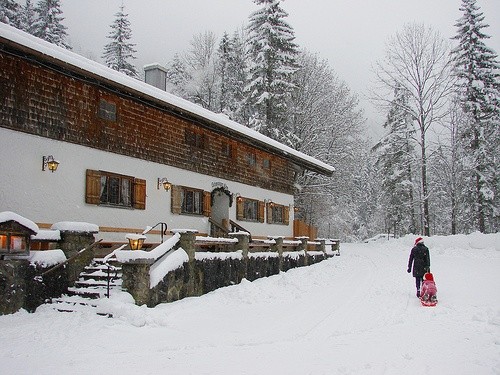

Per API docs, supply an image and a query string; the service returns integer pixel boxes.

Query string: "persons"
[405,236,431,297]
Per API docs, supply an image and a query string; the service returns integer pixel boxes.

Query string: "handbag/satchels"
[422,271,434,282]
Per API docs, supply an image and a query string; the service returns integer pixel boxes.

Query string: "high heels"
[417,290,421,298]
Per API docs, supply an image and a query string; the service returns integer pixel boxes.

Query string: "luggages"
[419,266,438,307]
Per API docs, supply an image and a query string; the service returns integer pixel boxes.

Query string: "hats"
[414,237,424,245]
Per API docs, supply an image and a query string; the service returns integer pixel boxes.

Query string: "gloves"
[407,267,412,274]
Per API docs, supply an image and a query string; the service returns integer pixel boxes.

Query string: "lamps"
[234,192,242,204]
[158,178,172,192]
[125,232,147,250]
[267,198,273,207]
[42,155,59,173]
[294,206,299,211]
